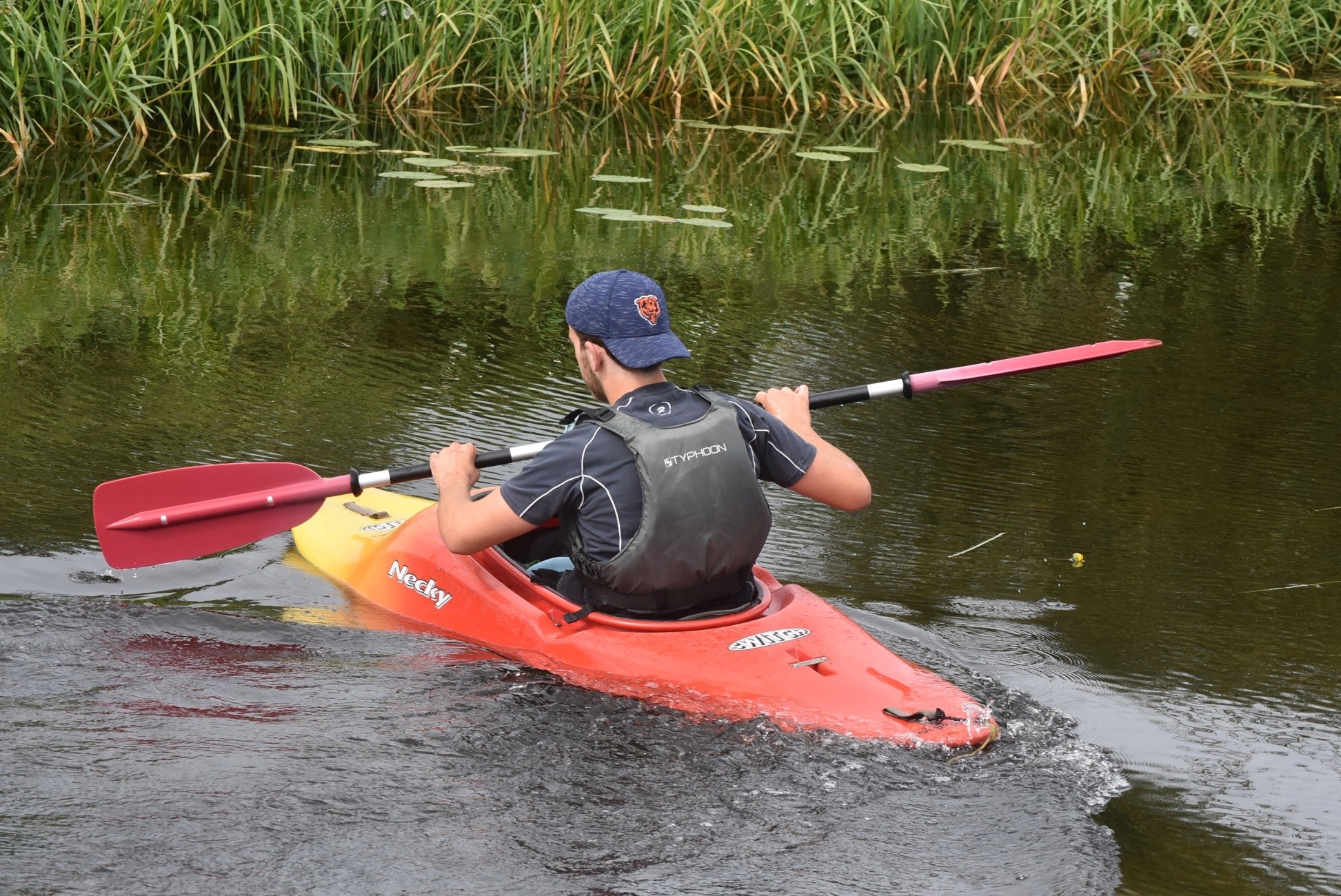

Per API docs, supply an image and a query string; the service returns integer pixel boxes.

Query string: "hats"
[567,268,691,369]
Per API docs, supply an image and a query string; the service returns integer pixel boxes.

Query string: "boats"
[289,486,1004,750]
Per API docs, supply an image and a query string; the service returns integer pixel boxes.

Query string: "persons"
[430,270,871,622]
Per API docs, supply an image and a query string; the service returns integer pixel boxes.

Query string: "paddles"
[90,336,1166,570]
